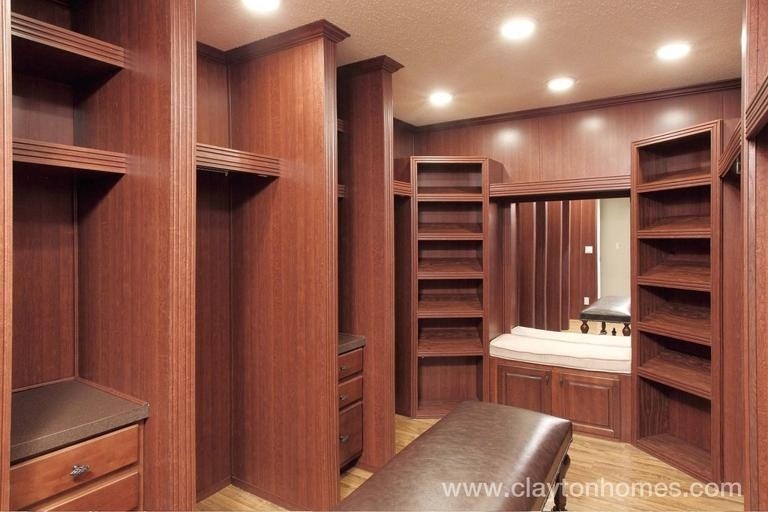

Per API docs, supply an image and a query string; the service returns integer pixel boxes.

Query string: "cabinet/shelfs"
[408,154,508,420]
[0,0,405,512]
[489,354,631,439]
[625,0,768,511]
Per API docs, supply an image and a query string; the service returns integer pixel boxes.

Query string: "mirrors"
[497,196,629,346]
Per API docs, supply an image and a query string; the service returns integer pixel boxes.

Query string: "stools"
[579,295,629,337]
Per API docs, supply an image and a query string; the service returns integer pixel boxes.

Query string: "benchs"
[316,394,580,511]
